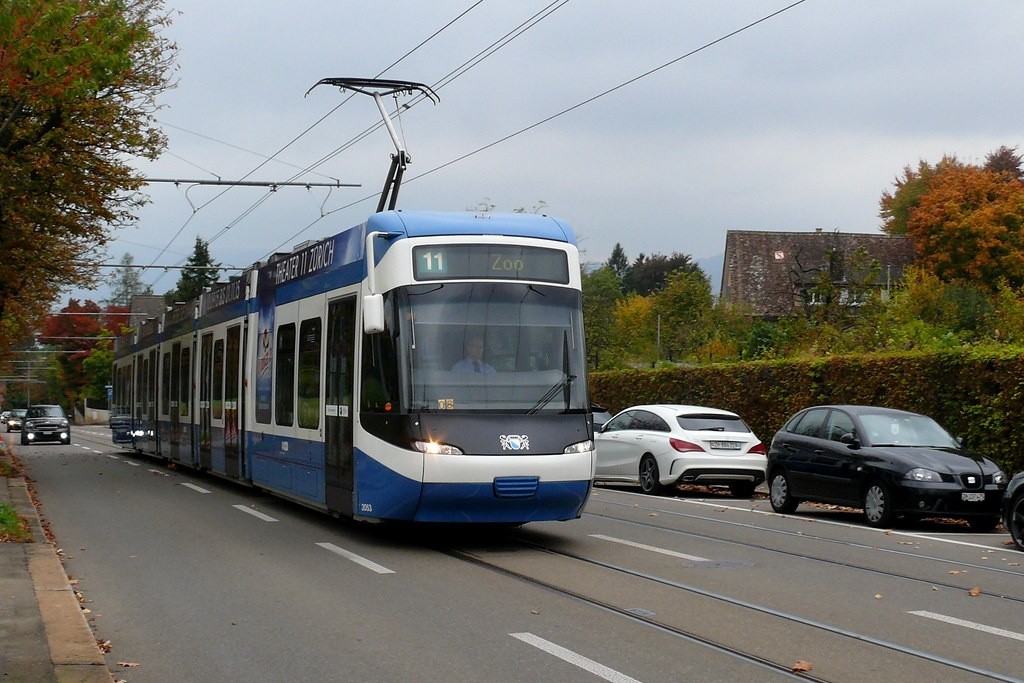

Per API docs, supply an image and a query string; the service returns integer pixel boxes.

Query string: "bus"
[111,76,595,534]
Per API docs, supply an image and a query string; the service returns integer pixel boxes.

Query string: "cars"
[1003,471,1024,552]
[592,403,769,495]
[766,405,1006,533]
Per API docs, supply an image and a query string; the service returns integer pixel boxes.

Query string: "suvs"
[0,405,72,446]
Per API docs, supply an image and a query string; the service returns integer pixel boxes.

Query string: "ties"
[473,361,481,373]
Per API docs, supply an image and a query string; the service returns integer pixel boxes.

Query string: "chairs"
[435,329,494,372]
[832,423,856,443]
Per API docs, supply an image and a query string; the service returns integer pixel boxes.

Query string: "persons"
[452,335,496,372]
[263,329,269,353]
